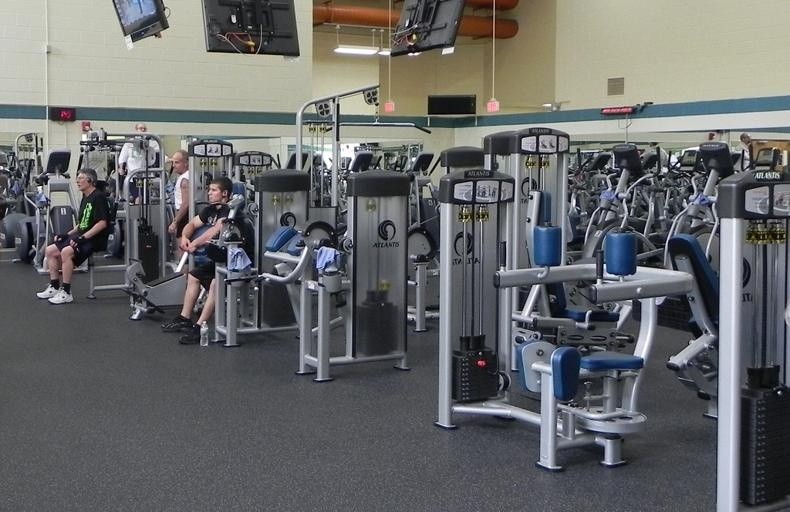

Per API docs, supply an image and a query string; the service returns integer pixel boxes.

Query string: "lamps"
[384,0,396,113]
[487,0,501,114]
[332,21,422,57]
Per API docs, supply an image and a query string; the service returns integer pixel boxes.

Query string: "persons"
[159,177,248,345]
[167,151,191,274]
[118,122,174,206]
[735,133,751,172]
[35,168,111,305]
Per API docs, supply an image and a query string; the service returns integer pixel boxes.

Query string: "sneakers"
[179,324,201,343]
[48,287,74,304]
[37,284,63,298]
[161,315,194,331]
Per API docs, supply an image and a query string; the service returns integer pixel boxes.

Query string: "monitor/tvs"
[390,0,465,56]
[112,0,169,43]
[200,0,300,57]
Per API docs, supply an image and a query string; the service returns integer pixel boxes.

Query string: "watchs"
[172,219,179,226]
